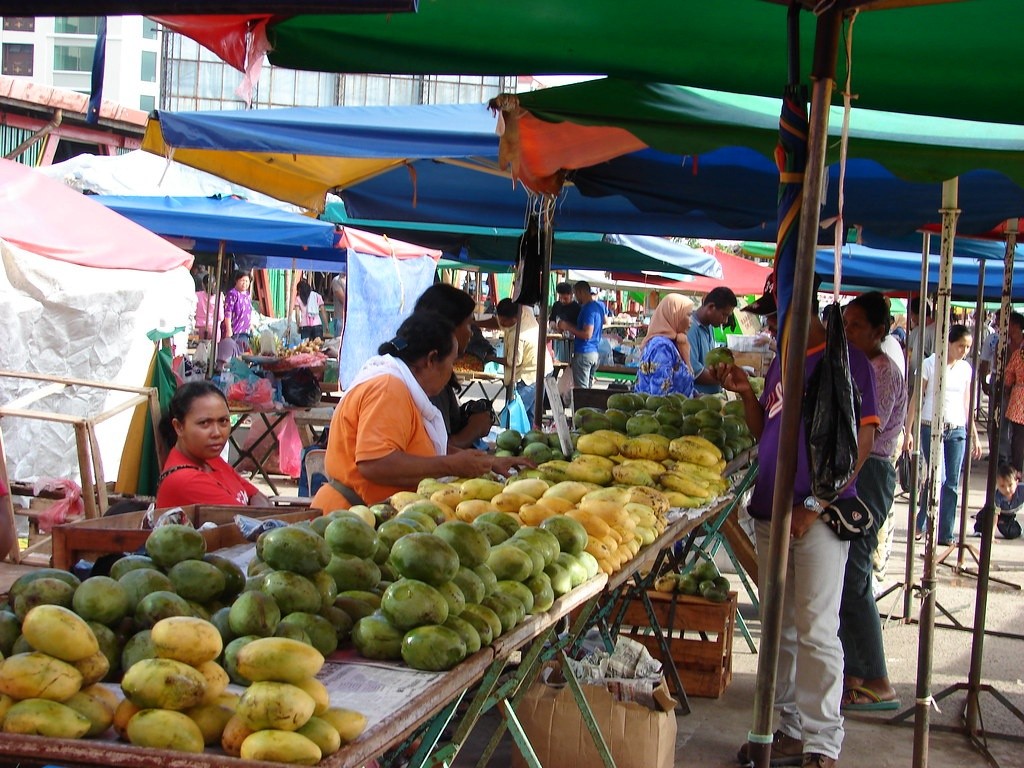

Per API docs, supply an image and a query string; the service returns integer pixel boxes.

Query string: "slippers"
[839,685,900,710]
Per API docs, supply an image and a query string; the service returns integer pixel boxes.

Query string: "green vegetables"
[249,336,279,356]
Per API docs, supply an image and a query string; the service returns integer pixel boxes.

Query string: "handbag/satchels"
[820,495,874,541]
[899,447,927,494]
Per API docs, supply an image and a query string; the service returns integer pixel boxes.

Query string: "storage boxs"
[570,576,738,699]
[506,661,677,768]
[27,481,323,572]
[725,333,774,376]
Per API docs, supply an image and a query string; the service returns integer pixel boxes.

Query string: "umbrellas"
[319,201,775,294]
[142,98,1024,432]
[734,238,1024,304]
[487,76,1024,768]
[99,194,442,376]
[149,1,1024,768]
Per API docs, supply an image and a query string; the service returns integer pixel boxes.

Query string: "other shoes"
[916,527,921,540]
[937,540,953,547]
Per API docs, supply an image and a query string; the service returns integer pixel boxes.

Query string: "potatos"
[285,336,322,355]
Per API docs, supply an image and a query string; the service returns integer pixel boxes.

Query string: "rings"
[790,534,793,536]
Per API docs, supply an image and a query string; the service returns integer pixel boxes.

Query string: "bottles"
[483,329,505,358]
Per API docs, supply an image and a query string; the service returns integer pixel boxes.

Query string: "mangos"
[641,559,730,602]
[0,502,598,764]
[385,395,757,577]
[706,347,734,370]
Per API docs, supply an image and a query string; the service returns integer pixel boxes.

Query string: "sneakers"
[800,753,837,768]
[737,729,803,768]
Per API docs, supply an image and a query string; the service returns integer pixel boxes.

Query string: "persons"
[463,274,475,294]
[192,263,253,356]
[294,272,346,339]
[483,280,617,431]
[633,293,696,398]
[685,287,737,394]
[155,380,273,507]
[414,283,493,450]
[310,314,535,516]
[880,297,1023,545]
[709,271,882,768]
[840,292,907,711]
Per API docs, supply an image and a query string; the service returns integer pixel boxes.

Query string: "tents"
[0,158,199,487]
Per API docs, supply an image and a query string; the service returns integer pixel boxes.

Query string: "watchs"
[804,496,823,514]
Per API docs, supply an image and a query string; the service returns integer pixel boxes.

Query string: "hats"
[740,271,822,316]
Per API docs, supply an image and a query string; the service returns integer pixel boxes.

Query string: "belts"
[921,419,965,430]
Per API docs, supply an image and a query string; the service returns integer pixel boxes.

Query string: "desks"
[0,495,738,768]
[228,406,335,495]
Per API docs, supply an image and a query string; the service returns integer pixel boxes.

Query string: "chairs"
[305,449,331,497]
